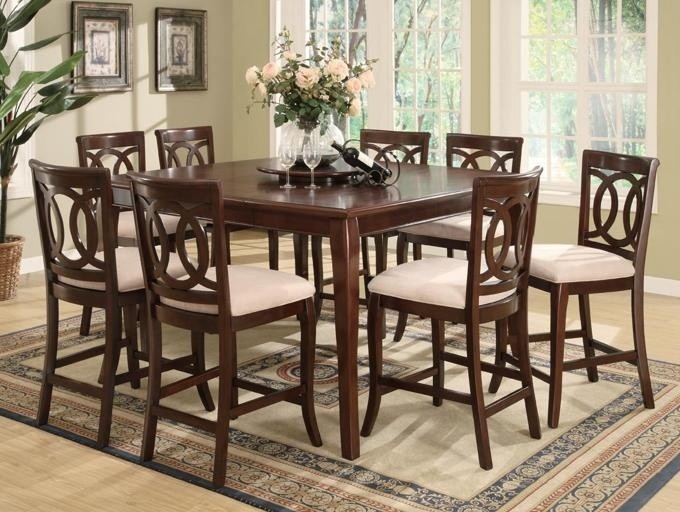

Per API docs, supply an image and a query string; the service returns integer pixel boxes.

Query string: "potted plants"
[0,0,99,302]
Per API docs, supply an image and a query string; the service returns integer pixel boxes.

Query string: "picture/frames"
[155,7,208,93]
[70,1,133,94]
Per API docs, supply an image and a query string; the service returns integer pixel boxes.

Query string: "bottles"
[329,140,392,182]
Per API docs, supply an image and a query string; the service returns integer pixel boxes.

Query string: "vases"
[276,114,345,167]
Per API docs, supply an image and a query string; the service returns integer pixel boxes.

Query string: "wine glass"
[300,123,323,190]
[273,127,299,190]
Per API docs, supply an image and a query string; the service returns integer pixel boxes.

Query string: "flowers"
[243,23,380,136]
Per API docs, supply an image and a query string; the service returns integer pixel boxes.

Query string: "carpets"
[0,297,680,512]
